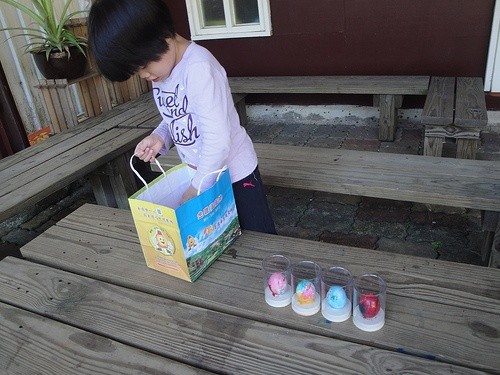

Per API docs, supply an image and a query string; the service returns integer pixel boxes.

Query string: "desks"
[0,203,500,375]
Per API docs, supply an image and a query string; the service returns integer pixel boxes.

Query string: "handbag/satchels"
[127,151,243,283]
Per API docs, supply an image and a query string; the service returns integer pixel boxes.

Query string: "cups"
[320,265,353,323]
[291,261,321,316]
[261,255,294,308]
[352,272,386,333]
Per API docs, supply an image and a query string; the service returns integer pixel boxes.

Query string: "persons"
[87,0,279,235]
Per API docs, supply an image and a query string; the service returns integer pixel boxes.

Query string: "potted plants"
[0,0,89,82]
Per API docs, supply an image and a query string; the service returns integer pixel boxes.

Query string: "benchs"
[0,89,248,224]
[421,76,489,159]
[150,143,500,269]
[226,75,430,142]
